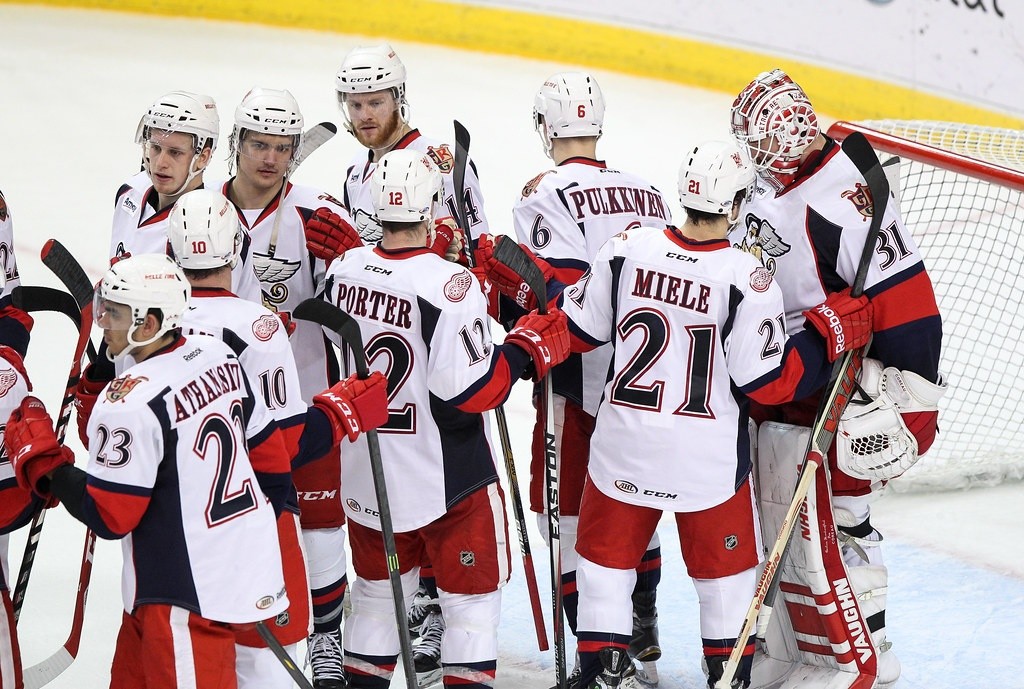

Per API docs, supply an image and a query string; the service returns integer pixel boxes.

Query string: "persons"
[0,43,571,689]
[699,69,942,689]
[512,71,664,685]
[479,141,873,689]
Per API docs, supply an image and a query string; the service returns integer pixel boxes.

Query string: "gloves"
[75,363,114,452]
[427,216,556,320]
[5,397,75,504]
[308,209,364,272]
[802,287,874,364]
[503,308,571,384]
[312,370,389,444]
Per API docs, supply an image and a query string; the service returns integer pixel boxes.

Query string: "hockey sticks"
[267,121,338,258]
[754,130,890,642]
[492,234,571,689]
[20,337,109,689]
[713,258,876,689]
[11,238,96,631]
[292,297,420,689]
[451,119,550,651]
[10,284,317,689]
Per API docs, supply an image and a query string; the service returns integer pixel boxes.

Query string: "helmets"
[369,149,446,225]
[532,70,607,150]
[167,189,244,270]
[134,90,219,176]
[678,140,758,225]
[232,84,304,148]
[333,42,409,109]
[92,253,191,346]
[729,69,818,196]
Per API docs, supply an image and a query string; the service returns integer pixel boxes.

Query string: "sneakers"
[701,654,752,689]
[617,654,643,688]
[550,647,627,689]
[628,590,662,688]
[302,587,446,689]
